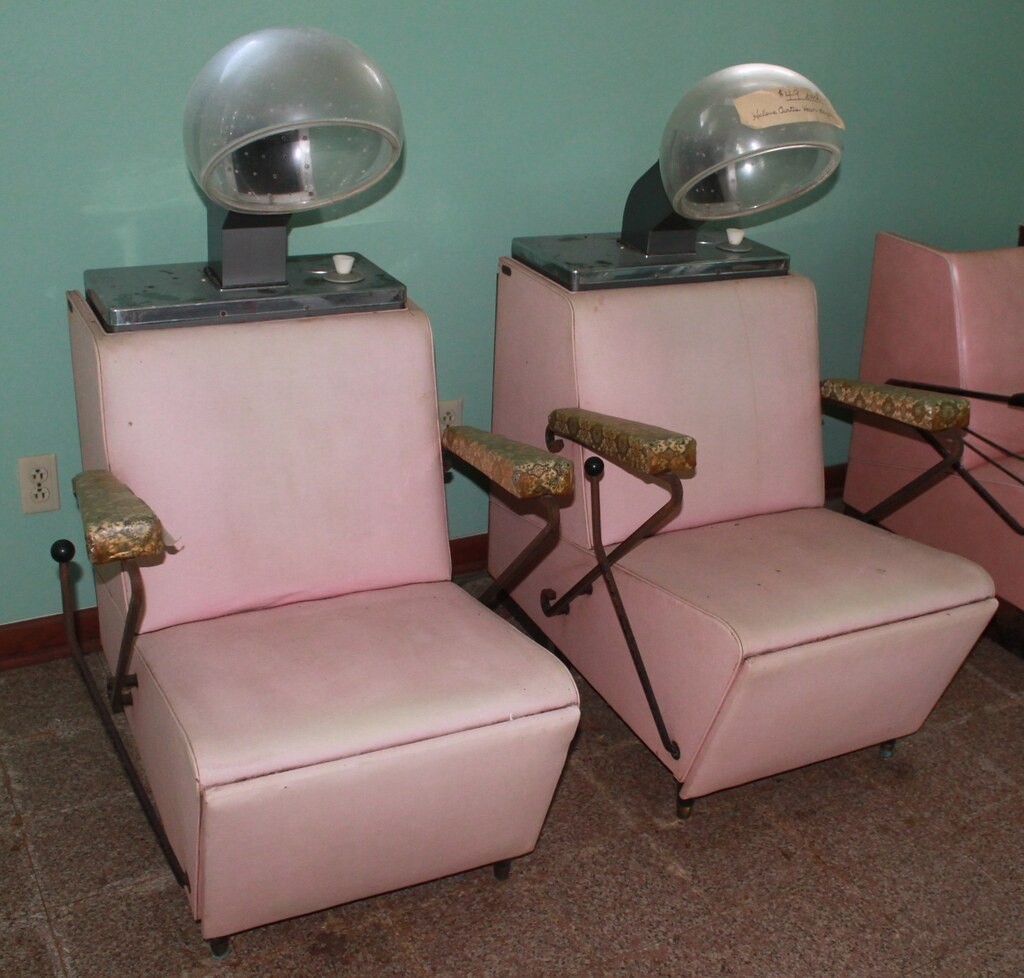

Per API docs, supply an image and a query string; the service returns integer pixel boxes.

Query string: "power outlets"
[438,400,463,436]
[16,453,63,516]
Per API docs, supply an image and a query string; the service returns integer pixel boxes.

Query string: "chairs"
[485,227,1002,820]
[57,253,588,956]
[838,229,1024,661]
